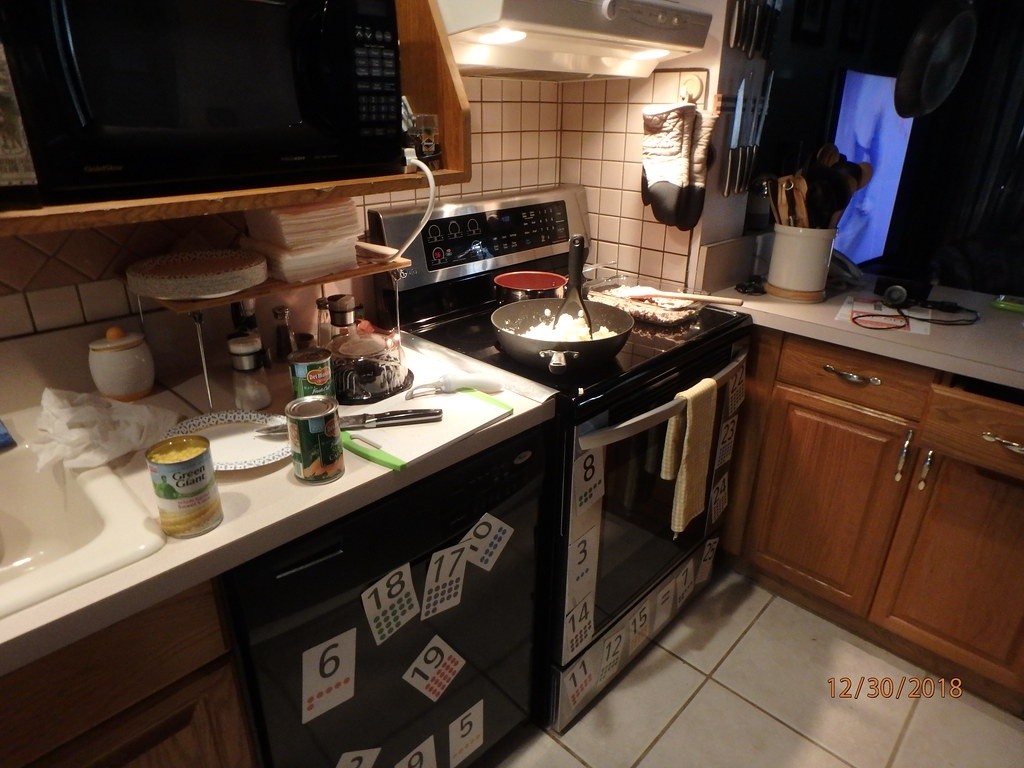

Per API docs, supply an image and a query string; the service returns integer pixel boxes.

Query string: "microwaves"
[0,0,402,206]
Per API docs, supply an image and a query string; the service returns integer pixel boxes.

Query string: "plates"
[125,250,269,302]
[157,410,293,472]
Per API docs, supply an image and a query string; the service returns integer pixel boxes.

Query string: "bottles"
[315,297,331,350]
[270,306,299,366]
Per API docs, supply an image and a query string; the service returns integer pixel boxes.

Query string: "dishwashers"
[213,422,554,768]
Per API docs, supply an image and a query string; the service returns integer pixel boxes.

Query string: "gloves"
[640,103,718,231]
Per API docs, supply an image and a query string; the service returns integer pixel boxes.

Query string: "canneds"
[143,435,224,539]
[287,347,337,400]
[284,394,345,485]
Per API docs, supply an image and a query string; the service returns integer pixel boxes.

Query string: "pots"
[894,0,987,119]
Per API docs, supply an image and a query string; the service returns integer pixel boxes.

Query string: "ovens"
[546,328,757,737]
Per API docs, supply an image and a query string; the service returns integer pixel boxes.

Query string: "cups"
[226,336,273,414]
[291,333,315,350]
[686,216,838,304]
[405,114,441,172]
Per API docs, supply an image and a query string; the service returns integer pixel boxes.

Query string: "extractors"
[432,0,713,85]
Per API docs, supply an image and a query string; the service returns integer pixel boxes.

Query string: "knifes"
[255,406,444,433]
[720,68,775,198]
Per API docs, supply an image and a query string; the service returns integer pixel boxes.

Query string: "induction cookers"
[367,182,755,403]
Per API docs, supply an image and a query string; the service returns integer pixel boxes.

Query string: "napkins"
[244,196,365,286]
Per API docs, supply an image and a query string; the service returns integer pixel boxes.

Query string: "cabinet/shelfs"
[0,570,254,768]
[718,324,1024,722]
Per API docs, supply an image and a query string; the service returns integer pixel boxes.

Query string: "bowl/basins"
[492,271,569,308]
[489,298,634,375]
[318,334,414,405]
